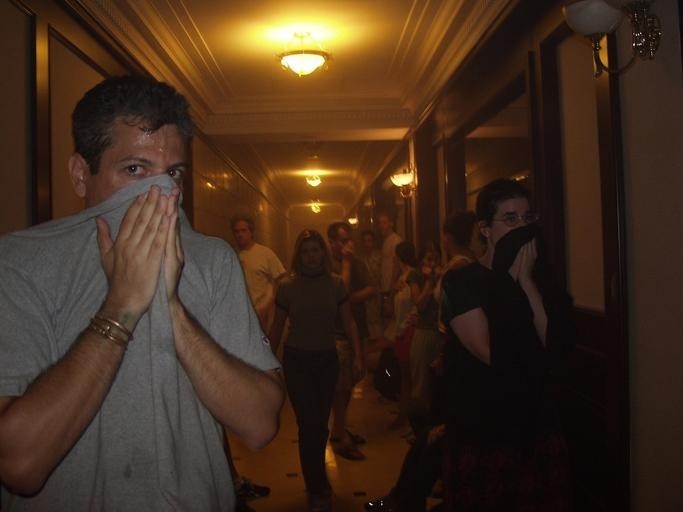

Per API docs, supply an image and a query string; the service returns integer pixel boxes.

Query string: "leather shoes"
[365,496,426,512]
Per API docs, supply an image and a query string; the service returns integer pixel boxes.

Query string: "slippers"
[329,431,363,461]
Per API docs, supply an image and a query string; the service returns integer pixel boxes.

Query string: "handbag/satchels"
[376,346,400,401]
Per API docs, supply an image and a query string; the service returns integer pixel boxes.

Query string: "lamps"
[389,168,417,200]
[559,0,664,79]
[306,176,326,187]
[275,50,332,78]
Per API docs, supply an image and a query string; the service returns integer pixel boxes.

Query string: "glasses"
[486,211,541,227]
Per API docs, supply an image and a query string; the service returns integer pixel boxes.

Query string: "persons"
[327,221,374,461]
[1,72,286,511]
[360,209,477,511]
[441,179,574,510]
[268,229,365,498]
[231,212,285,331]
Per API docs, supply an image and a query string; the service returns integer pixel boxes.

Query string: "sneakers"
[234,477,270,512]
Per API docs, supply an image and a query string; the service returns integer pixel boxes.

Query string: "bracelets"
[87,309,133,346]
